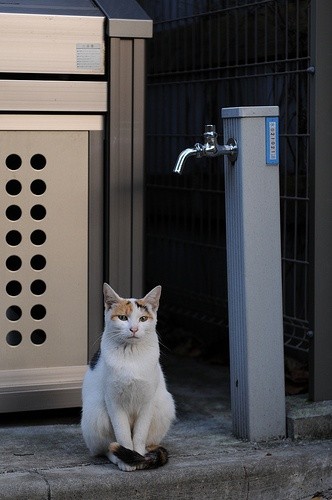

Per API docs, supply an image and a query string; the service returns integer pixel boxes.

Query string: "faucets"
[172,123,238,178]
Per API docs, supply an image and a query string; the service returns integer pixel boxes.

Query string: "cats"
[80,283,177,473]
